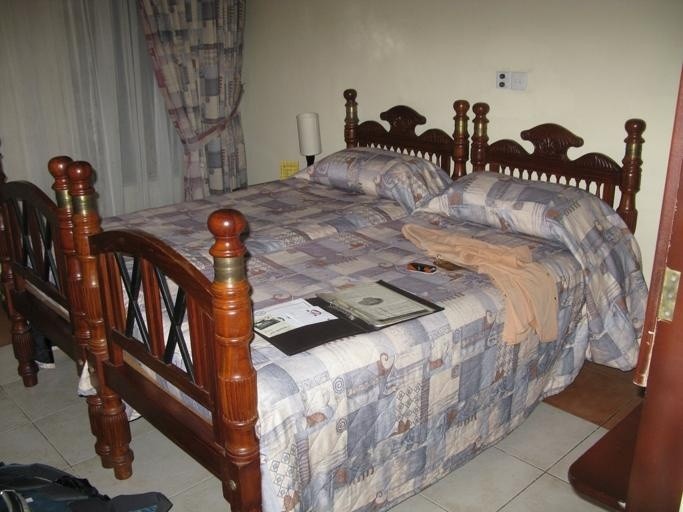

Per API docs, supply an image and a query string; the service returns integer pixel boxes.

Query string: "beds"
[0,87,470,468]
[66,103,648,512]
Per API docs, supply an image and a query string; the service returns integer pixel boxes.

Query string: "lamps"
[296,112,322,168]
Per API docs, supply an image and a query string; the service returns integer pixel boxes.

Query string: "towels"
[401,223,561,346]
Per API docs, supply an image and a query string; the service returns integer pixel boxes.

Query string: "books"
[313,278,438,329]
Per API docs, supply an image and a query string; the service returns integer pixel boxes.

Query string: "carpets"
[541,359,645,430]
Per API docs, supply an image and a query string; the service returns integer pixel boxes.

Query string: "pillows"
[412,171,613,248]
[293,147,455,215]
